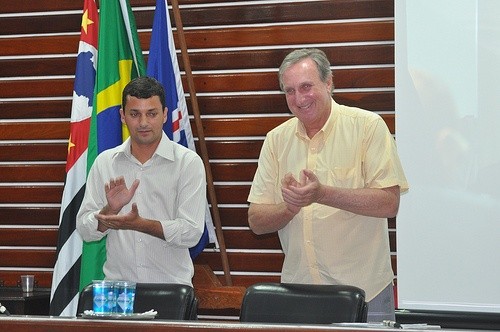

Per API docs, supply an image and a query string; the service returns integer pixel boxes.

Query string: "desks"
[0,286,51,315]
[0,315,500,332]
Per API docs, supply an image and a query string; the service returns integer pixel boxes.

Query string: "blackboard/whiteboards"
[395,0,499,312]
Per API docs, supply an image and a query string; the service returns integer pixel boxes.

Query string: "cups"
[92,280,114,313]
[115,282,136,312]
[21,275,34,292]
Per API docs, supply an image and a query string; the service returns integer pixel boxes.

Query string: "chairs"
[239,281,368,324]
[75,282,197,319]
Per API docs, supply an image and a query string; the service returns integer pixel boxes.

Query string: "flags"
[48,0,220,318]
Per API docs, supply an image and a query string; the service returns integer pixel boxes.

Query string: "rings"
[109,222,110,225]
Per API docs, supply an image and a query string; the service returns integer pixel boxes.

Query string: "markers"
[382,319,400,327]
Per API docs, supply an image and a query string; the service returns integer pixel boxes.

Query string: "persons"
[76,78,207,288]
[246,48,408,323]
